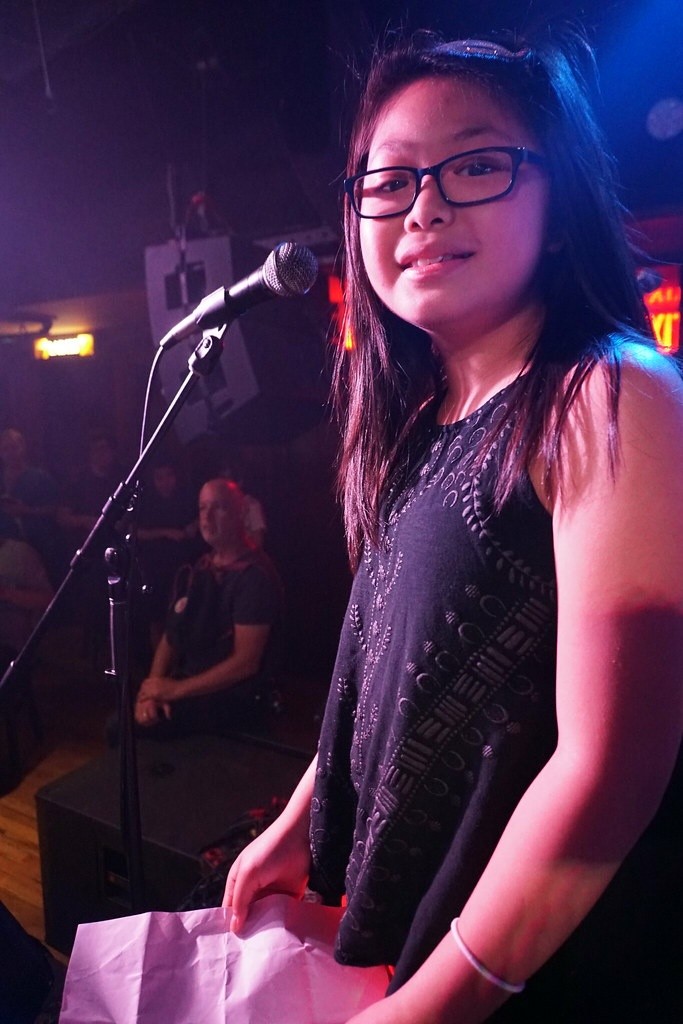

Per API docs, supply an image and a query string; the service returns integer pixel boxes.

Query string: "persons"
[130,480,274,733]
[222,34,683,1024]
[2,422,268,688]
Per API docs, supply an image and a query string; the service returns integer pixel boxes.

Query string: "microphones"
[160,243,319,350]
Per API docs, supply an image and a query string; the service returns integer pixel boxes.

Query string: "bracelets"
[448,916,522,995]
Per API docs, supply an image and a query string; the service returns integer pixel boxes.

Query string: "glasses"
[343,146,550,220]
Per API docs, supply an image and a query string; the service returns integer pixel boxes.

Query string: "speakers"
[36,719,318,955]
[142,231,332,444]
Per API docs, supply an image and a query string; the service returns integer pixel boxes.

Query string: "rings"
[142,713,148,717]
